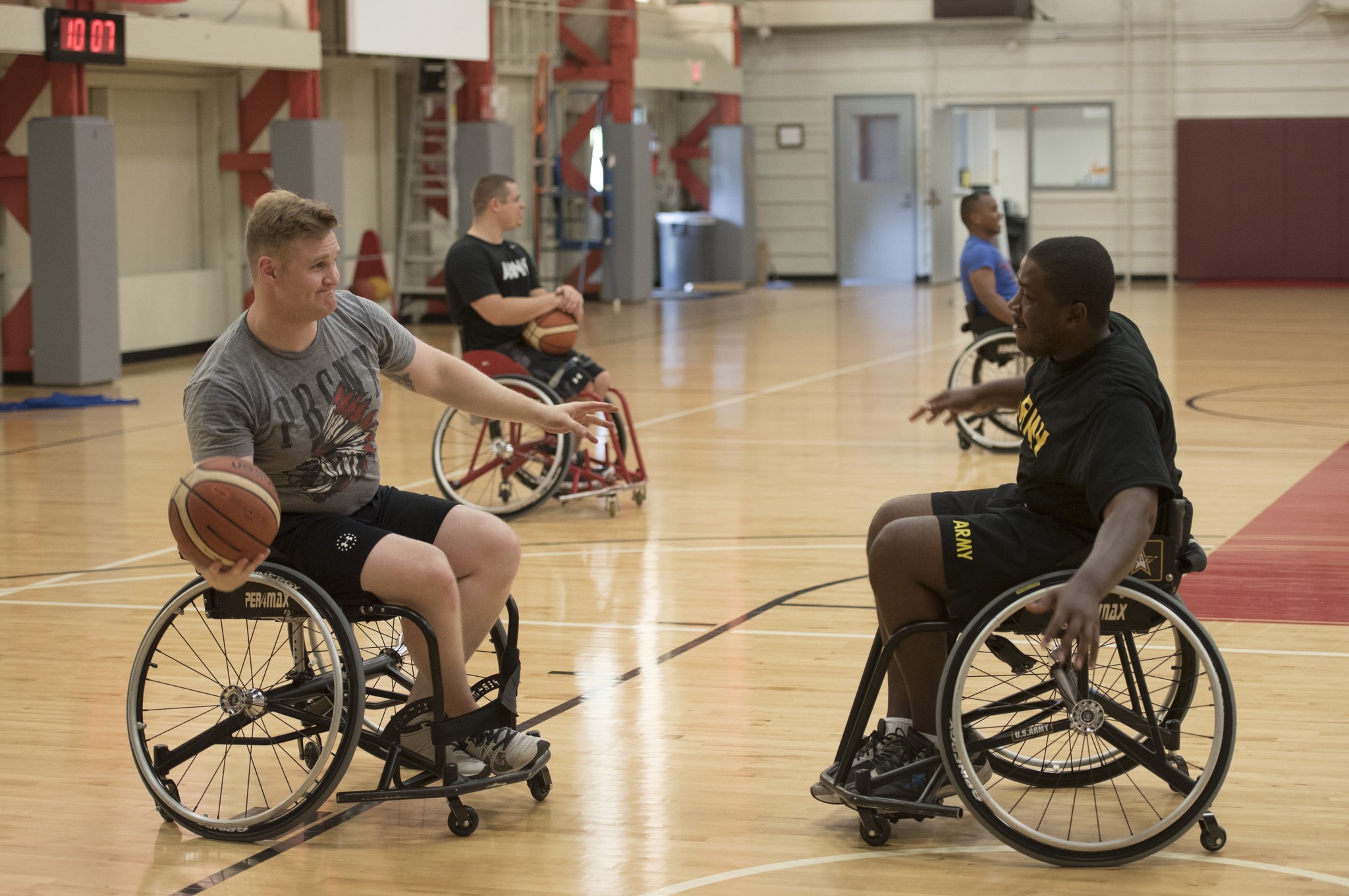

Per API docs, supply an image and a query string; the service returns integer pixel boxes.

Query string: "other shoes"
[540,463,597,490]
[566,448,617,479]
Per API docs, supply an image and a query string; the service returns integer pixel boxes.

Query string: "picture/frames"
[775,122,807,152]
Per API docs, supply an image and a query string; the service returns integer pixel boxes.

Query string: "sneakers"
[400,720,491,780]
[462,727,550,776]
[811,718,907,805]
[839,726,992,813]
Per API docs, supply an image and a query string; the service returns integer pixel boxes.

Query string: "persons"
[181,189,620,779]
[810,236,1176,815]
[444,175,617,491]
[960,190,1023,327]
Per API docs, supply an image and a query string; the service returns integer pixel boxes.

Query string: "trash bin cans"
[656,212,716,292]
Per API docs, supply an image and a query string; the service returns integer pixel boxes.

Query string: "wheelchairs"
[124,558,555,844]
[946,302,1041,456]
[430,325,651,521]
[818,465,1238,868]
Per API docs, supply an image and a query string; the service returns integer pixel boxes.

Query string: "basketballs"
[522,309,579,355]
[168,457,282,572]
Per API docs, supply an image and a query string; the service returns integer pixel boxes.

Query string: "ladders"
[532,86,621,306]
[392,57,460,312]
